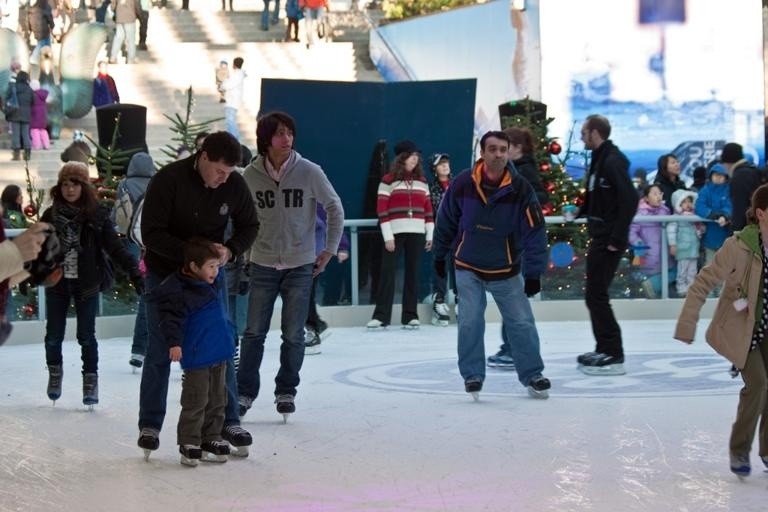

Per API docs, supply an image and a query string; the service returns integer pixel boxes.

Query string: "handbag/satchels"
[4,94,19,116]
[296,11,304,19]
[39,266,64,288]
[110,192,131,237]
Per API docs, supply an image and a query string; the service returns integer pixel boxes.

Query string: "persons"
[0,111,349,466]
[576,115,640,376]
[216,57,248,140]
[674,184,767,482]
[1,1,149,160]
[368,0,377,8]
[367,126,551,402]
[629,144,768,298]
[157,1,330,44]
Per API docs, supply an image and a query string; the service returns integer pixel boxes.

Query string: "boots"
[82,370,99,404]
[12,147,31,160]
[47,363,63,399]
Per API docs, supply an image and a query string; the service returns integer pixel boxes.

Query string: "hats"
[58,160,90,186]
[430,151,449,165]
[393,140,422,154]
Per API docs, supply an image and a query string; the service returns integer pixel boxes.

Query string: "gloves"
[129,265,145,295]
[20,279,36,296]
[710,210,729,221]
[434,260,446,278]
[40,222,60,262]
[524,279,541,297]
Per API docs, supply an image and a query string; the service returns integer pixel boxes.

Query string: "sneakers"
[577,350,624,365]
[366,295,458,327]
[304,320,327,346]
[129,354,144,367]
[530,373,551,390]
[180,443,203,459]
[203,440,231,455]
[238,394,253,416]
[223,426,252,446]
[465,376,483,392]
[488,349,516,367]
[138,427,161,451]
[275,394,295,413]
[729,452,751,474]
